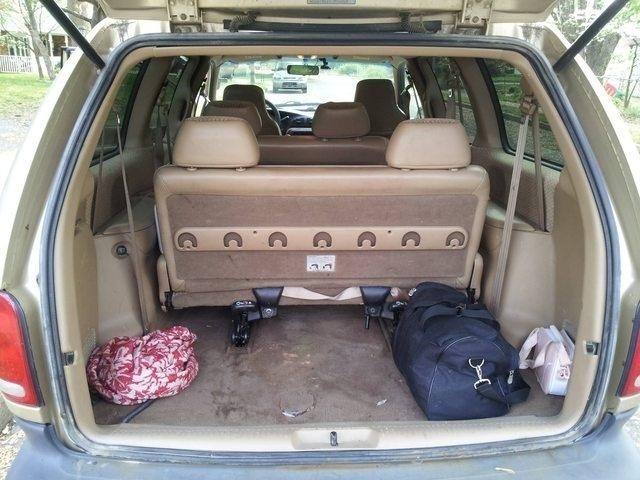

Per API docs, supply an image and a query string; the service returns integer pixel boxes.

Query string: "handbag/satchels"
[532,327,575,396]
[392,282,519,421]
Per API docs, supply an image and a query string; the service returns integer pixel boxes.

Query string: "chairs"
[152,78,492,315]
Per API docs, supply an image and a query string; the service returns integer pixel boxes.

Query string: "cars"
[271,61,309,93]
